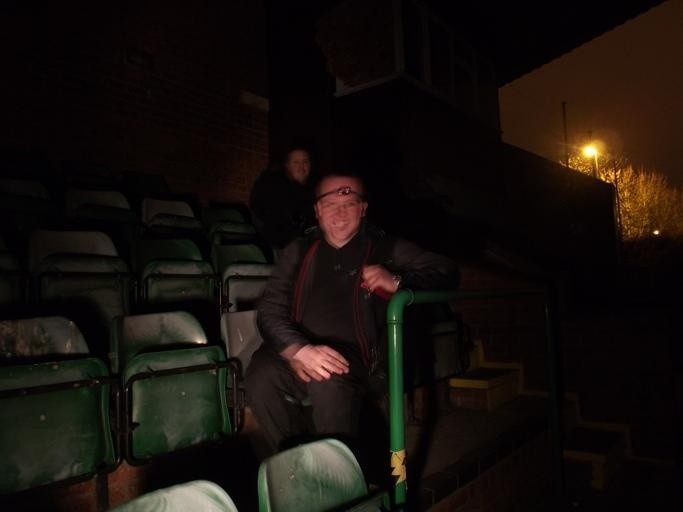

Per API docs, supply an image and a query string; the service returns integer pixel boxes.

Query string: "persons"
[243,173,457,464]
[245,135,331,265]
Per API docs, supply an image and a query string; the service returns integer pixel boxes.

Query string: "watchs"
[393,272,401,292]
